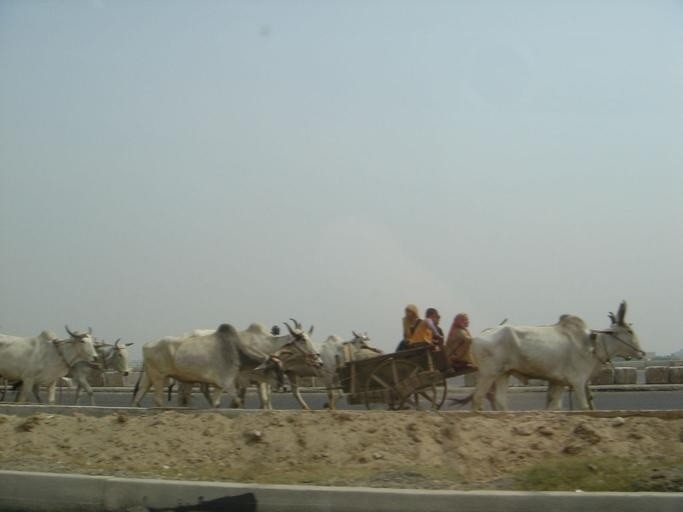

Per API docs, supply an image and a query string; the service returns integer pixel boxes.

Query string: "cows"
[446,299,648,412]
[131,318,382,409]
[13,337,135,409]
[0,324,100,409]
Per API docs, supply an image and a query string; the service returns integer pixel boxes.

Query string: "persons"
[430,314,444,347]
[444,313,473,375]
[267,322,286,394]
[396,303,436,350]
[407,308,443,352]
